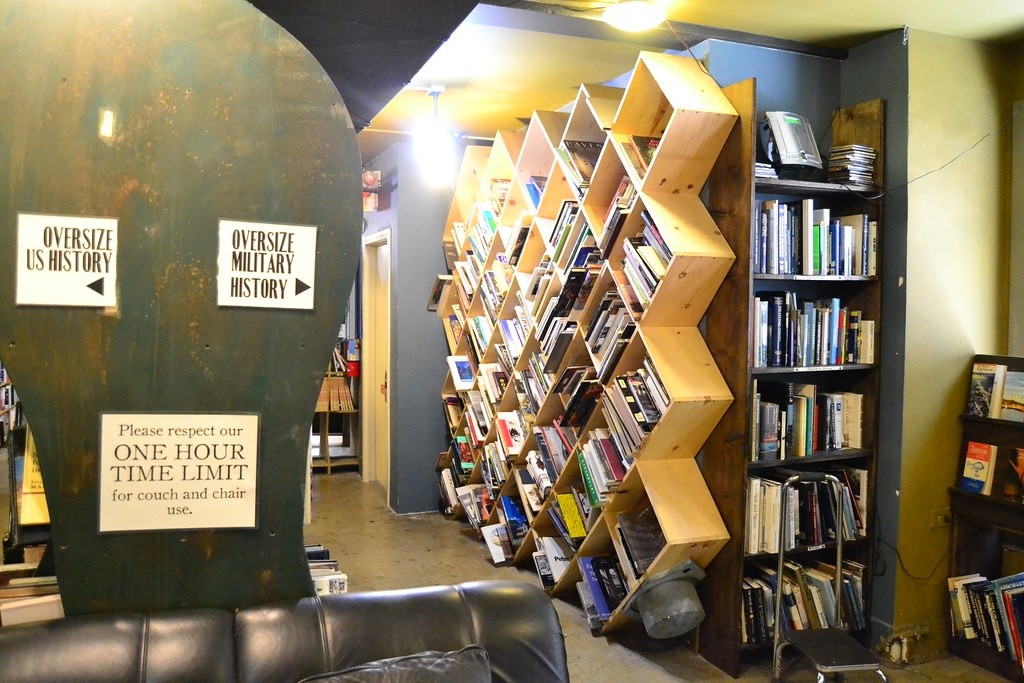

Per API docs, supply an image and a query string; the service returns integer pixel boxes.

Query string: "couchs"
[0,577,575,683]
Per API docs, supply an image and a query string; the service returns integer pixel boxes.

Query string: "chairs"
[768,469,890,683]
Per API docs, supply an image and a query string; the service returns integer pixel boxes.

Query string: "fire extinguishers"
[347,340,360,377]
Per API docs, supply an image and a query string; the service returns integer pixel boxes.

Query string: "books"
[946,571,1024,667]
[0,544,61,606]
[961,361,1024,424]
[304,543,348,596]
[745,145,879,644]
[362,170,382,211]
[0,361,23,449]
[441,138,671,635]
[961,441,1024,509]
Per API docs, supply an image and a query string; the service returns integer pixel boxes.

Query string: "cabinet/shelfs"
[296,42,1024,683]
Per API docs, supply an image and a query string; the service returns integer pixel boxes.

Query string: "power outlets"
[930,506,952,532]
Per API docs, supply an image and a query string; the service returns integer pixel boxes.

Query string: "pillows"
[298,643,492,683]
